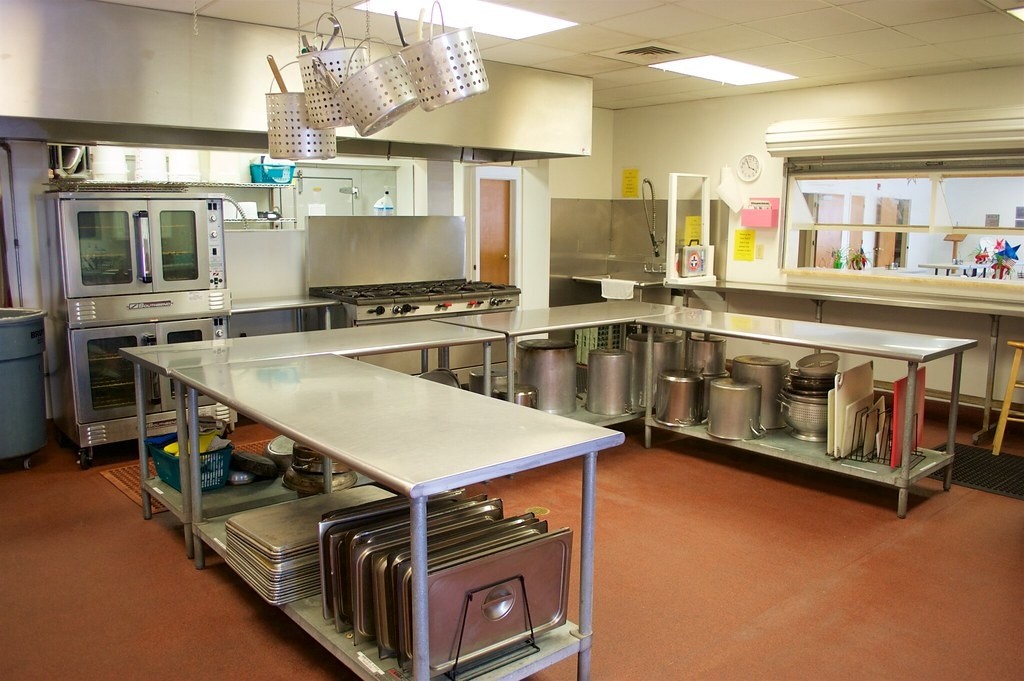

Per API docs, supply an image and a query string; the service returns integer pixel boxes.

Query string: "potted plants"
[829,246,873,271]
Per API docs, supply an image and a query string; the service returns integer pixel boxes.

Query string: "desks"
[116,297,978,681]
[918,263,992,278]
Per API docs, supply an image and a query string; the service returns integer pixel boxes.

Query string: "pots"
[775,373,836,443]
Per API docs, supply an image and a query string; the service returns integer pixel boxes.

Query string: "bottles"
[373,191,395,216]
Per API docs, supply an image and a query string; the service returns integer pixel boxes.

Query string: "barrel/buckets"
[626,334,687,413]
[515,339,577,414]
[688,336,728,377]
[730,355,791,429]
[585,349,633,415]
[652,370,705,428]
[708,379,767,440]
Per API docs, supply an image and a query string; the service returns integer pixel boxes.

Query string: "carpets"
[926,441,1024,500]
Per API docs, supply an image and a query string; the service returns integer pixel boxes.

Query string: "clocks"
[737,154,762,183]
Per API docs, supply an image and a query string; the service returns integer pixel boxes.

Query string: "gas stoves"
[309,266,521,324]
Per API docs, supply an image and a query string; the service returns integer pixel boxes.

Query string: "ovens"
[40,192,237,448]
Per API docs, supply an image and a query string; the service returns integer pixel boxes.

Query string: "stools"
[991,339,1024,455]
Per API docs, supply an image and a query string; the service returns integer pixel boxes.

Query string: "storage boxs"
[144,432,236,493]
[250,164,296,184]
